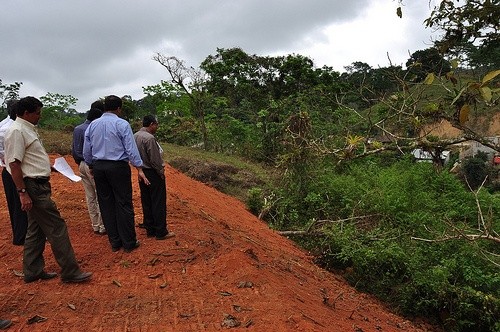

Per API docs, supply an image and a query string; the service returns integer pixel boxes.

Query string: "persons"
[133,115,175,240]
[0,96,93,283]
[72,94,151,253]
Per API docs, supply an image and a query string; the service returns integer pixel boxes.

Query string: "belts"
[24,176,49,181]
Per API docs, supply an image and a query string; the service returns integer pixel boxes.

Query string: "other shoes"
[124,243,139,253]
[94,231,99,234]
[112,246,122,252]
[99,231,107,235]
[0,319,13,330]
[156,232,175,240]
[13,241,24,245]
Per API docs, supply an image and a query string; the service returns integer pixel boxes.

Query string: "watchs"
[17,187,28,193]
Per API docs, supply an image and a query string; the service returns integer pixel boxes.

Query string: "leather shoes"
[24,270,57,282]
[62,272,93,283]
